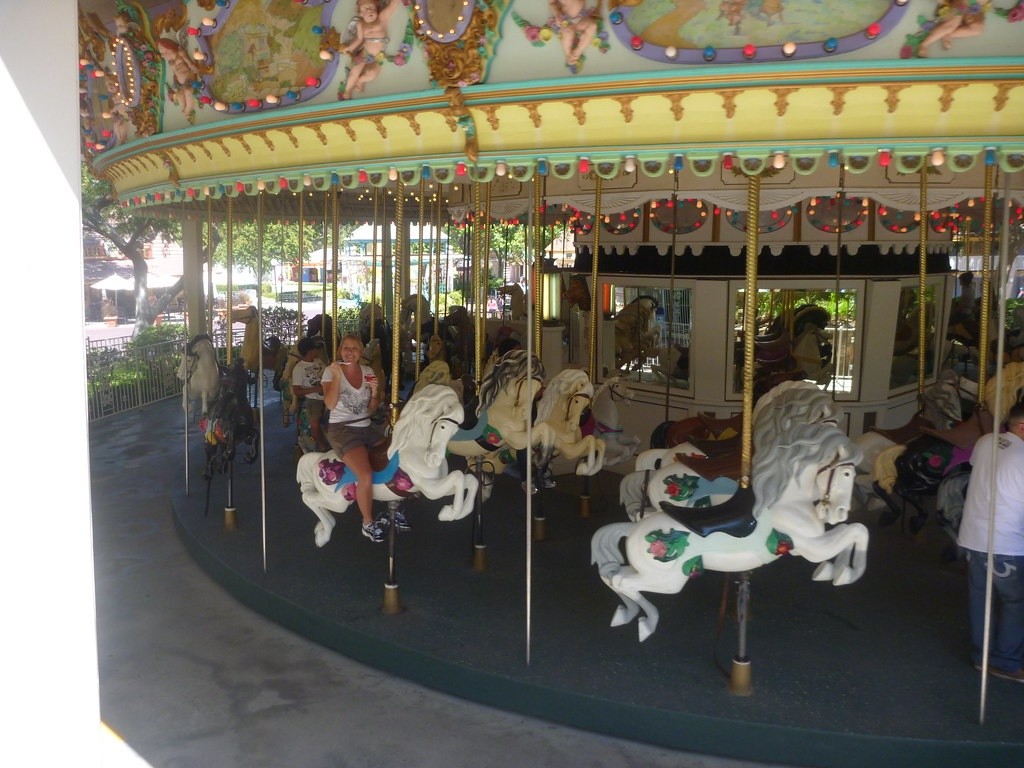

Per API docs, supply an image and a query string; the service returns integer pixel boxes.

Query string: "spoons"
[335,362,351,365]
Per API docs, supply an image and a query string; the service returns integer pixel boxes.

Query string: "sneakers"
[362,509,412,543]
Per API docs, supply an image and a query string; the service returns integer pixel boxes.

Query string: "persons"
[953,402,1024,683]
[292,337,327,444]
[321,331,411,544]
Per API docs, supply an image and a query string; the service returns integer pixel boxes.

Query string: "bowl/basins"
[365,374,376,383]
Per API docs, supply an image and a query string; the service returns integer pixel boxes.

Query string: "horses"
[650,371,808,449]
[296,384,480,548]
[202,358,258,479]
[578,377,642,466]
[278,291,492,454]
[636,380,821,471]
[175,334,229,419]
[232,305,288,388]
[893,303,936,354]
[590,422,869,642]
[740,323,835,390]
[617,390,847,529]
[560,272,591,311]
[413,349,557,469]
[466,369,606,503]
[947,292,999,353]
[615,295,661,372]
[733,304,831,365]
[495,284,523,321]
[848,369,1020,537]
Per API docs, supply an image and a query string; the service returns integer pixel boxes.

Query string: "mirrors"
[603,283,692,393]
[732,289,857,398]
[885,283,938,397]
